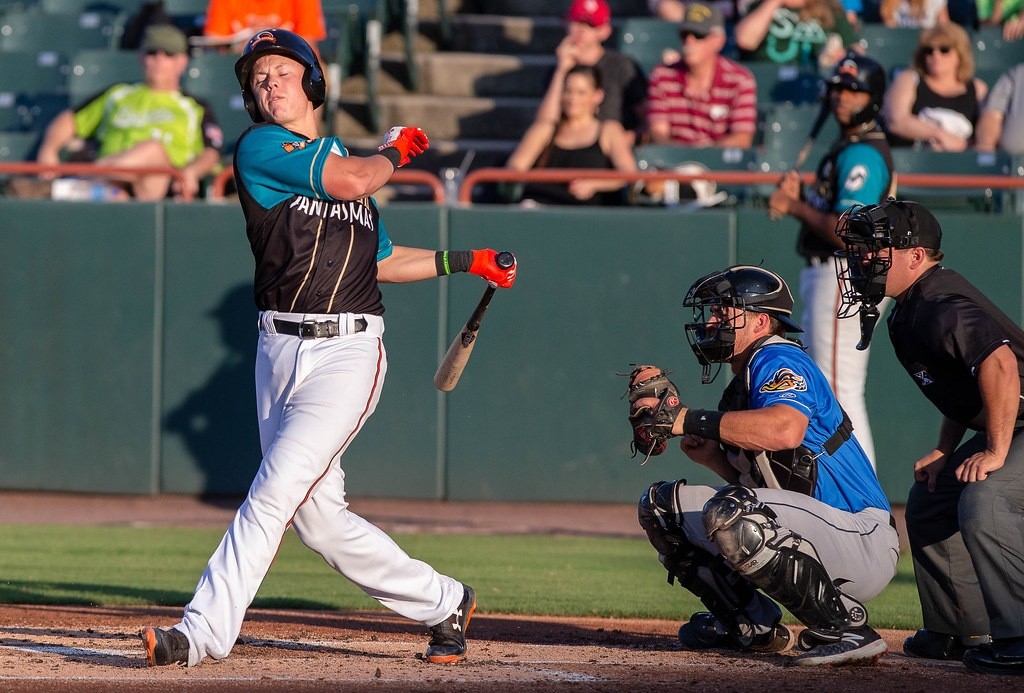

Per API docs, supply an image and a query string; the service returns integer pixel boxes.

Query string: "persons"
[0,0,1024,214]
[833,195,1024,674]
[140,29,517,666]
[770,51,896,478]
[623,263,901,666]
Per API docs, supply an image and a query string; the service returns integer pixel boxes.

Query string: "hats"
[566,0,612,26]
[143,23,186,54]
[855,204,942,248]
[678,2,725,34]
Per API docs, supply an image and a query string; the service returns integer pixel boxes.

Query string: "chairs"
[510,0,1024,209]
[0,0,457,204]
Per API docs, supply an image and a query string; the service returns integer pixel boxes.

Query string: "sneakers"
[787,623,888,667]
[143,625,188,668]
[678,615,795,653]
[424,582,477,662]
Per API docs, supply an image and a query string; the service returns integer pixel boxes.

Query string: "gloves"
[376,125,430,168]
[469,248,517,289]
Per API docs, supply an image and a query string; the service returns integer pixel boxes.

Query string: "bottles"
[51,178,120,203]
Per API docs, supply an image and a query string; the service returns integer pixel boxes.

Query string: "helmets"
[235,29,326,123]
[710,264,806,333]
[825,55,886,94]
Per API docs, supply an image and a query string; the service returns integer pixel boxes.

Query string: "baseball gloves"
[628,364,680,456]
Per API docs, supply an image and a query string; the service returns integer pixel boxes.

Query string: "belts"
[272,317,367,338]
[889,514,900,538]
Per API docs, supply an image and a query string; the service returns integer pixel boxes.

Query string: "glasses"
[679,29,706,39]
[922,44,956,54]
[145,48,174,56]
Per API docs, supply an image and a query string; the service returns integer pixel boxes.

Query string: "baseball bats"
[769,89,833,221]
[432,250,515,391]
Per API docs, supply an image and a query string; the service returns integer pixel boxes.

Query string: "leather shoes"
[903,629,992,660]
[962,643,1024,674]
